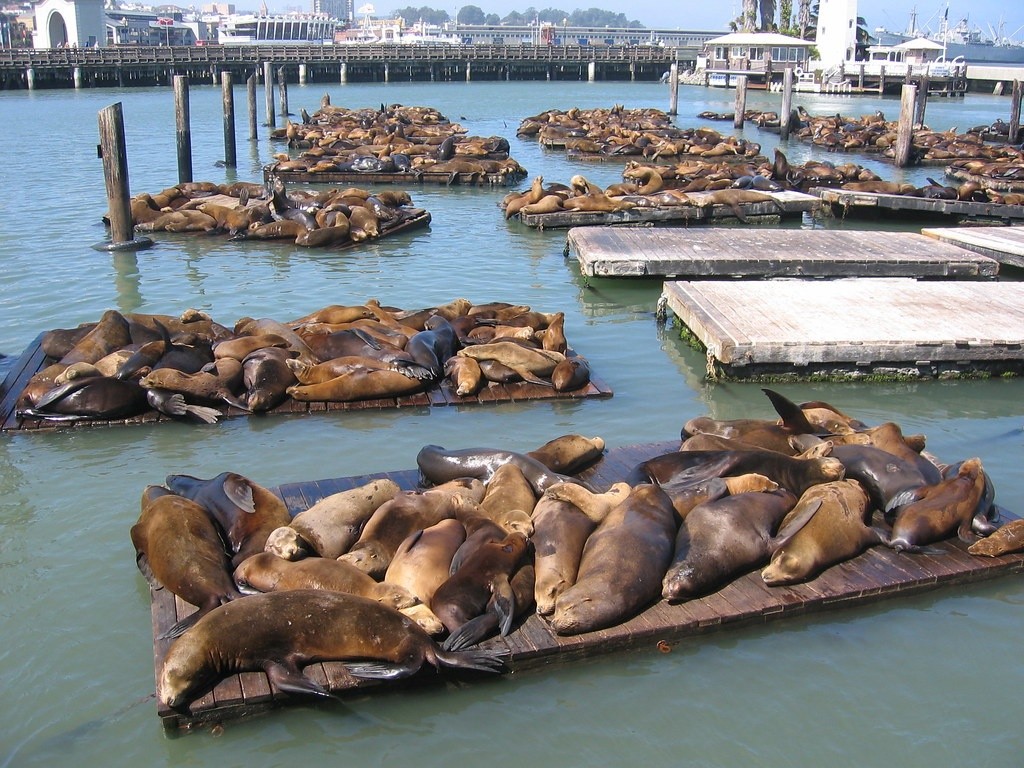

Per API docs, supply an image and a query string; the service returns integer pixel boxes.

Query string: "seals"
[14,297,1024,709]
[110,102,1024,248]
